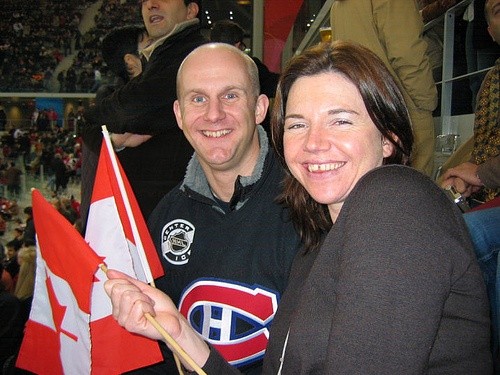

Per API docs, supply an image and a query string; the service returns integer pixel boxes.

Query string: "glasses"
[236,40,247,51]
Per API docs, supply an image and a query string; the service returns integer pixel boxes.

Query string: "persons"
[0,0,143,341]
[444,0,500,341]
[417,0,456,94]
[463,2,480,113]
[102,24,150,80]
[330,0,437,177]
[146,42,304,375]
[83,0,202,218]
[211,22,243,50]
[105,39,500,375]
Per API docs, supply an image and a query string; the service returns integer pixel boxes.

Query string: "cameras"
[446,184,471,214]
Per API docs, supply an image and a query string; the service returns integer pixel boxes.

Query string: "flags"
[15,189,102,375]
[84,138,164,375]
